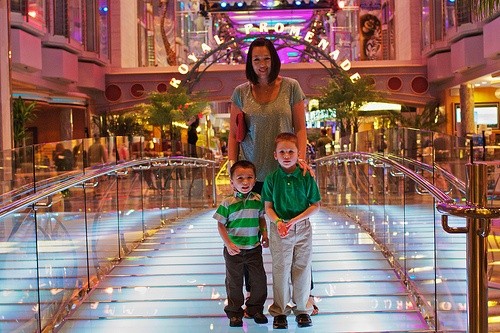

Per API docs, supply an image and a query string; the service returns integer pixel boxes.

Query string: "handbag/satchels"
[235,111,246,142]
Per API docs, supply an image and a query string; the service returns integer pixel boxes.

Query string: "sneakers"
[246,307,268,325]
[273,314,289,329]
[229,317,244,327]
[295,313,312,327]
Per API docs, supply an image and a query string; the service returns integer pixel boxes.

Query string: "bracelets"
[273,218,282,225]
[299,159,306,163]
[261,228,267,231]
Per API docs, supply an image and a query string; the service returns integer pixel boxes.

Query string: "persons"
[261,132,322,329]
[305,127,334,164]
[227,38,319,320]
[421,131,460,196]
[212,160,270,327]
[37,132,188,194]
